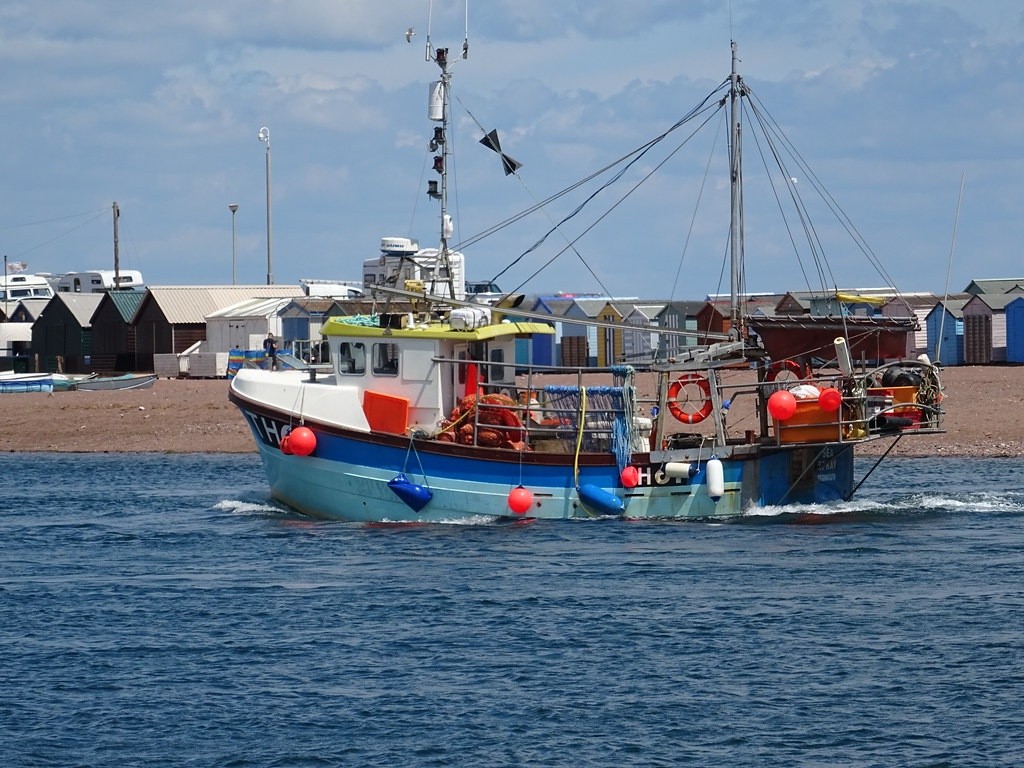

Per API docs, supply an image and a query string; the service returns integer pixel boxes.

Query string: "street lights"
[257,126,276,285]
[229,204,240,286]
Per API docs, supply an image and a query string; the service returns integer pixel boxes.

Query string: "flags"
[7,262,27,274]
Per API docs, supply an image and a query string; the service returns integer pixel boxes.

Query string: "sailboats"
[229,0,951,530]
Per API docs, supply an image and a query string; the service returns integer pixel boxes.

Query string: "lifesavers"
[766,360,803,384]
[667,374,712,424]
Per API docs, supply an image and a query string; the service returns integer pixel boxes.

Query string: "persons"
[263,333,277,370]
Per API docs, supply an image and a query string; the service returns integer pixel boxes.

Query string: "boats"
[70,372,161,392]
[0,367,78,392]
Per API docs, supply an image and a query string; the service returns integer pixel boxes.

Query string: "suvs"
[465,280,505,300]
